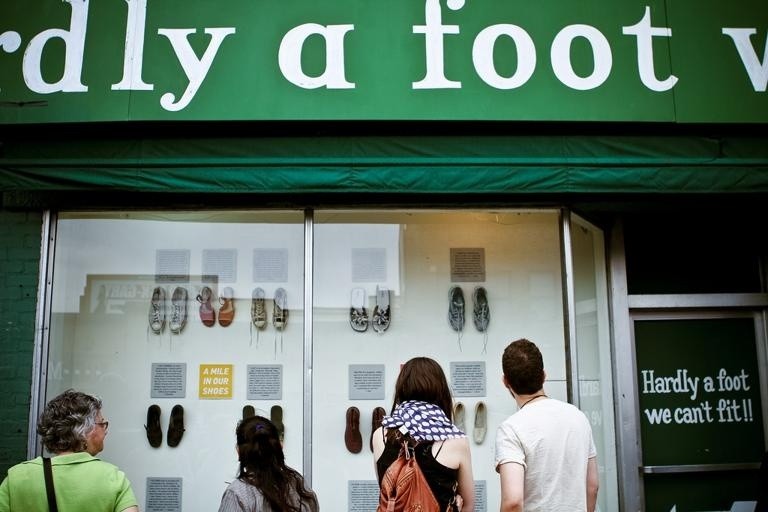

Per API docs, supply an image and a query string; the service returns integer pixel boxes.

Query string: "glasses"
[91,421,109,431]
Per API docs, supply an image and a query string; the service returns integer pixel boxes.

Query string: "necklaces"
[519,394,548,409]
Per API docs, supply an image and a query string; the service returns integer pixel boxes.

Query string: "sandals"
[454,401,489,445]
[350,282,392,334]
[196,285,235,328]
[145,403,186,448]
[241,405,284,449]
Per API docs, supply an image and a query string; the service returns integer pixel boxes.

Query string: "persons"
[0,388,139,511]
[492,336,601,512]
[372,355,479,512]
[218,417,320,511]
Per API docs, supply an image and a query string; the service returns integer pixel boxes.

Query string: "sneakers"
[247,286,289,329]
[148,285,189,334]
[448,284,490,331]
[344,407,386,455]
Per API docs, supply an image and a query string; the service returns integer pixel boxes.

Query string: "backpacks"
[376,442,441,512]
